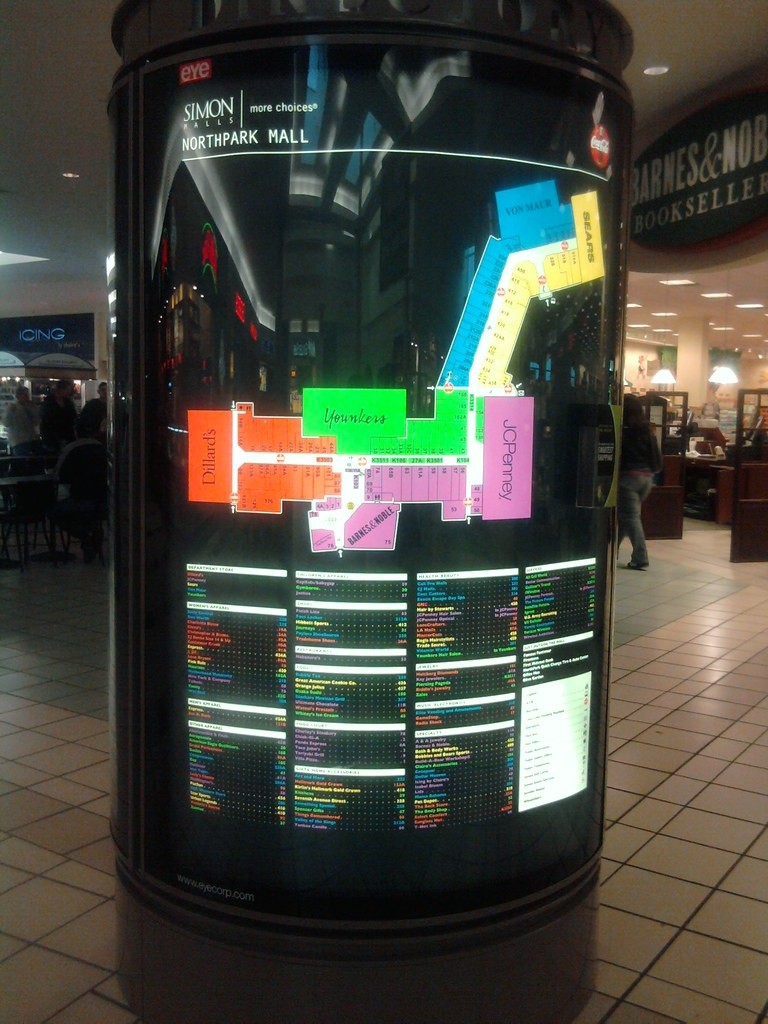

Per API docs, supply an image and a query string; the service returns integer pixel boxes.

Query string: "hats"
[55,378,74,390]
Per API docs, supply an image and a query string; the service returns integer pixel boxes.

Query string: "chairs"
[0,445,108,573]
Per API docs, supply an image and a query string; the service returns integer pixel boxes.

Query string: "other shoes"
[628,561,649,570]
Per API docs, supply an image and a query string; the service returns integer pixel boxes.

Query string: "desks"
[0,475,54,563]
[666,452,768,523]
[0,455,57,461]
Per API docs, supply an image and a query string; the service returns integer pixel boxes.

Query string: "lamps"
[709,271,738,383]
[651,273,676,384]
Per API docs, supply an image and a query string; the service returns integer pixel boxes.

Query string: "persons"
[39,380,77,448]
[80,381,108,443]
[3,386,40,455]
[618,393,662,569]
